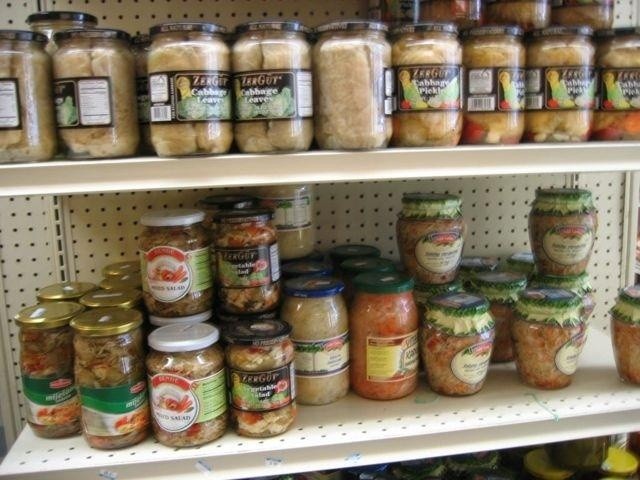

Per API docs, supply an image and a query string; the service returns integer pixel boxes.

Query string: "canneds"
[473,190,596,390]
[329,193,493,401]
[12,260,149,450]
[611,286,640,388]
[367,0,640,149]
[139,185,348,449]
[240,432,640,480]
[0,12,230,165]
[229,18,393,154]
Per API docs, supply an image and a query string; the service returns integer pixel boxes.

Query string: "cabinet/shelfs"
[0,0,640,479]
